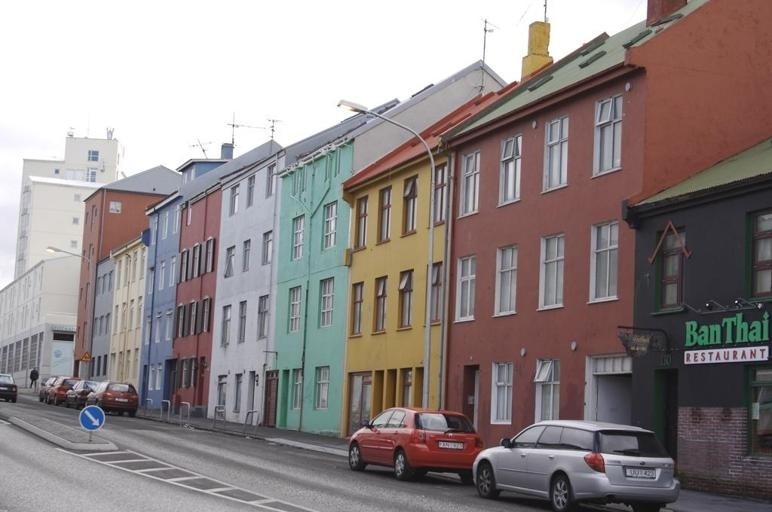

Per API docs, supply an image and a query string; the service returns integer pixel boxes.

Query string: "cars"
[39,373,140,417]
[474,419,678,510]
[0,371,17,404]
[350,408,480,483]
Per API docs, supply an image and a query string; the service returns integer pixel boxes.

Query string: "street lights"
[45,244,93,377]
[338,97,436,408]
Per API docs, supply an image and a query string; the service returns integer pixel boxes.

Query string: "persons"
[30,367,38,388]
